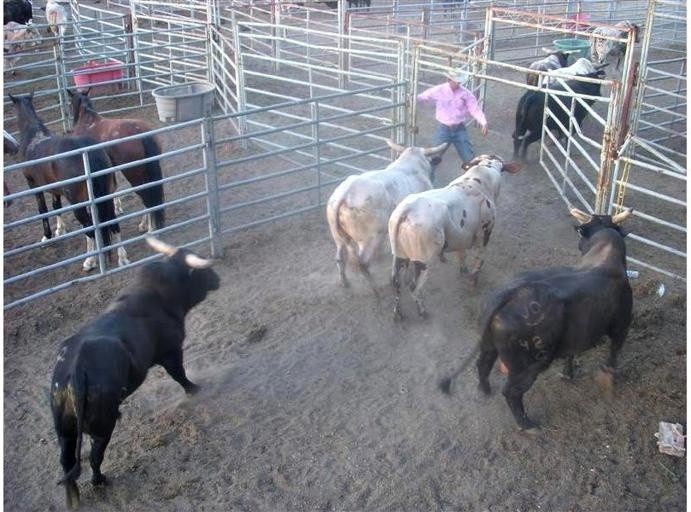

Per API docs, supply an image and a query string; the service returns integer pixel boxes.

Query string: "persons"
[417,71,487,161]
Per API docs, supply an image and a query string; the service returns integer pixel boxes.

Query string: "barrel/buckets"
[71,63,123,95]
[554,39,590,67]
[152,81,214,123]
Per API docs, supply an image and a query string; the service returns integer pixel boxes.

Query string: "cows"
[387,152,523,326]
[436,206,636,437]
[591,19,646,70]
[325,139,450,292]
[5,1,69,75]
[512,51,609,164]
[48,233,220,512]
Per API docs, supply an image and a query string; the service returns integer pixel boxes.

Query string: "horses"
[65,85,165,238]
[7,86,130,274]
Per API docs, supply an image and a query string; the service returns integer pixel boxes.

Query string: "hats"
[443,70,468,82]
